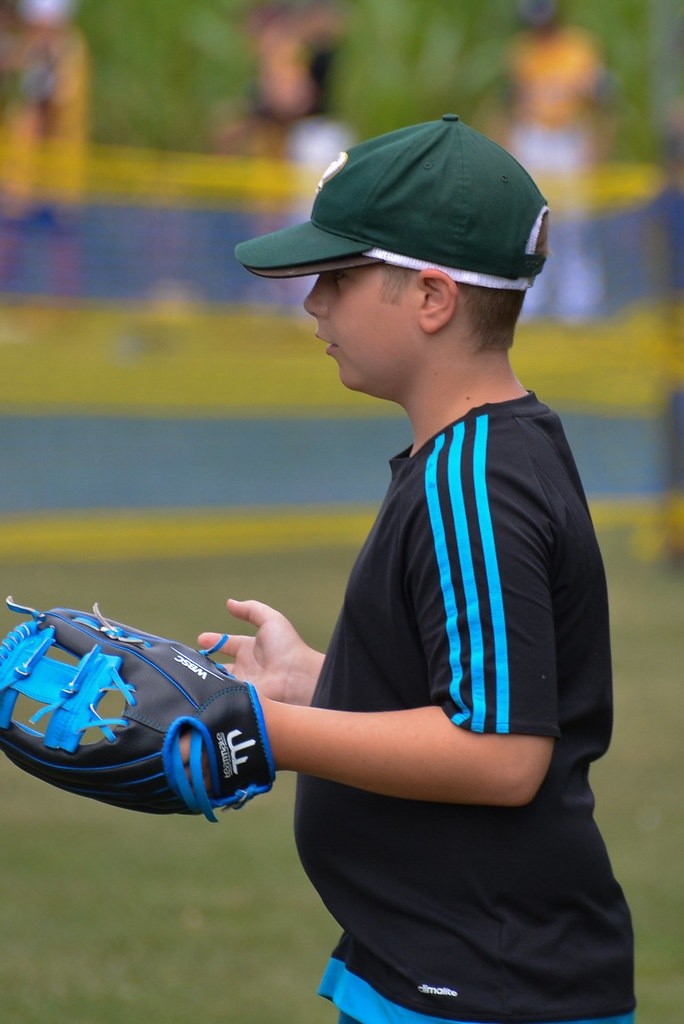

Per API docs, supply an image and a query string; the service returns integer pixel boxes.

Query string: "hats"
[234,112,551,293]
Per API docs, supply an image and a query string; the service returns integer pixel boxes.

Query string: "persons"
[215,0,348,315]
[0,112,637,1024]
[499,1,608,313]
[0,0,89,297]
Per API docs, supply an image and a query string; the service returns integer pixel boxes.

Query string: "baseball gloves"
[0,593,278,824]
